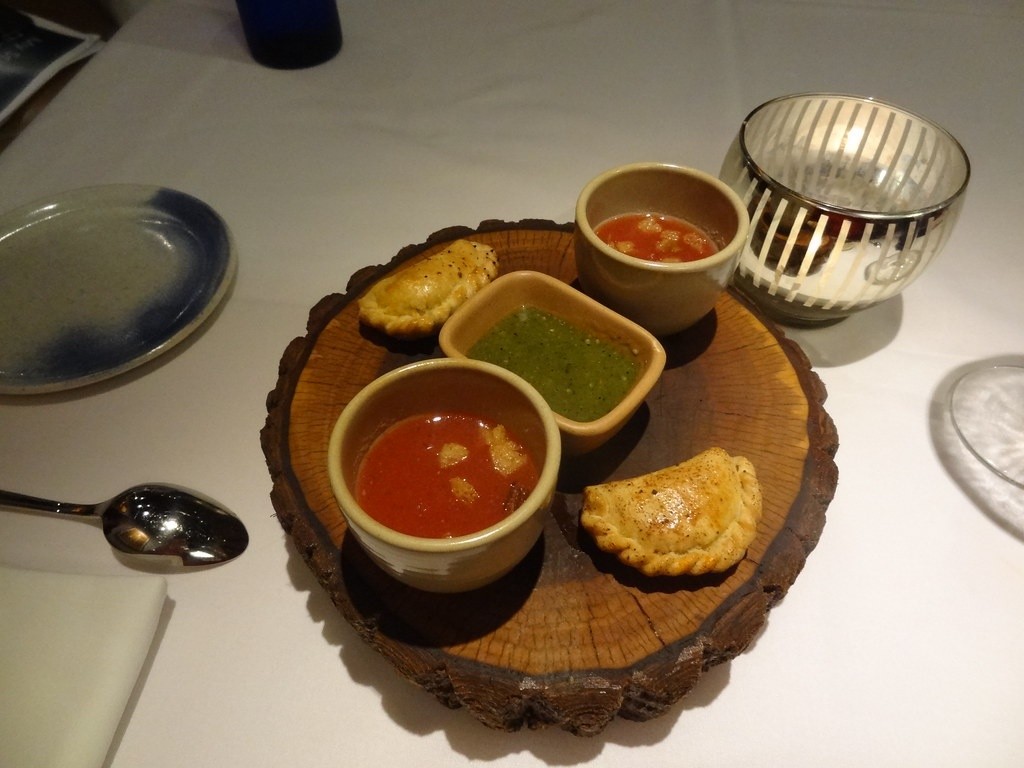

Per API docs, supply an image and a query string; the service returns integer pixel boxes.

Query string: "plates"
[0,184,237,394]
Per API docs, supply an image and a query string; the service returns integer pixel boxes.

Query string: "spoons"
[0,482,249,567]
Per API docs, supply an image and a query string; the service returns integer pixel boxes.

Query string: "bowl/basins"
[575,160,751,337]
[326,356,562,593]
[720,94,971,330]
[438,271,667,455]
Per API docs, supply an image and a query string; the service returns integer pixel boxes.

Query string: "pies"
[579,447,763,576]
[357,238,499,333]
